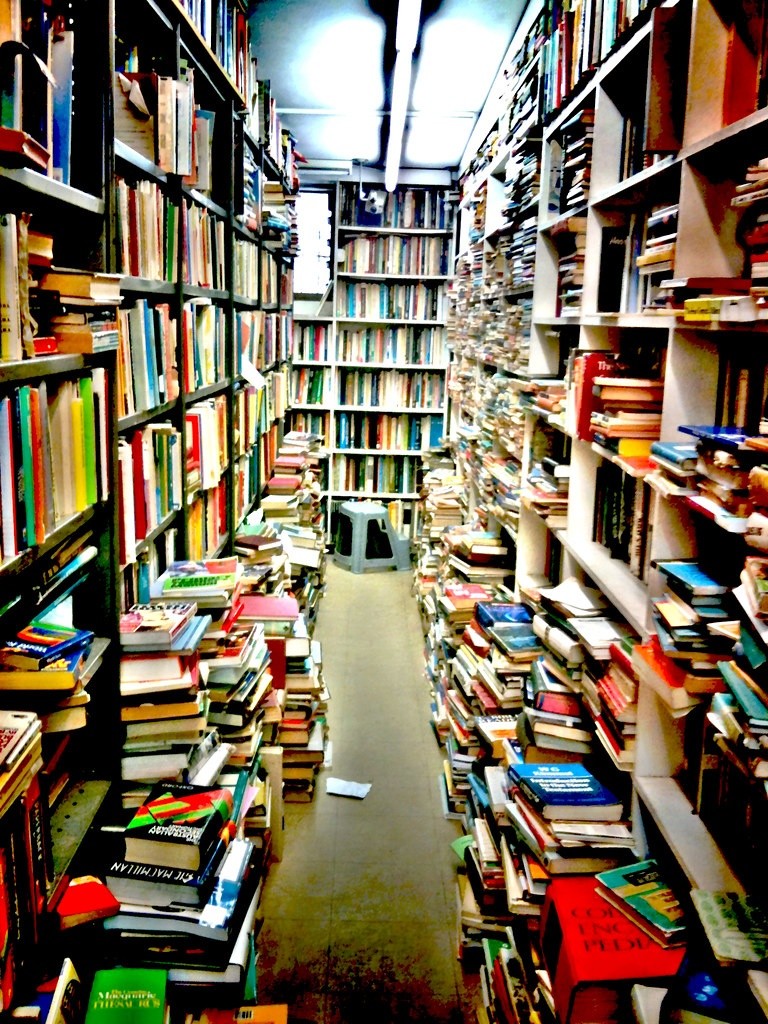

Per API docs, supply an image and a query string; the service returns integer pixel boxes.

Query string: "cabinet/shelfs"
[0,0,768,1023]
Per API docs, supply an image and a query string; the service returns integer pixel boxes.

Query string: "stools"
[334,503,410,573]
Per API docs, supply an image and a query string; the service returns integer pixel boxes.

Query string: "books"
[0,0,768,1024]
